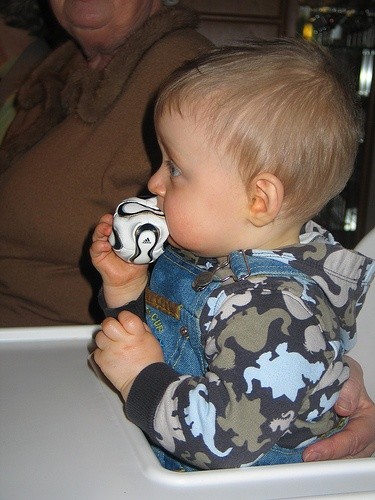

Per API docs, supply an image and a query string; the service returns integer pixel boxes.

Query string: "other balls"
[106,191,170,268]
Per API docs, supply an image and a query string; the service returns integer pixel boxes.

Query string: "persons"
[0,0,375,464]
[89,30,364,472]
